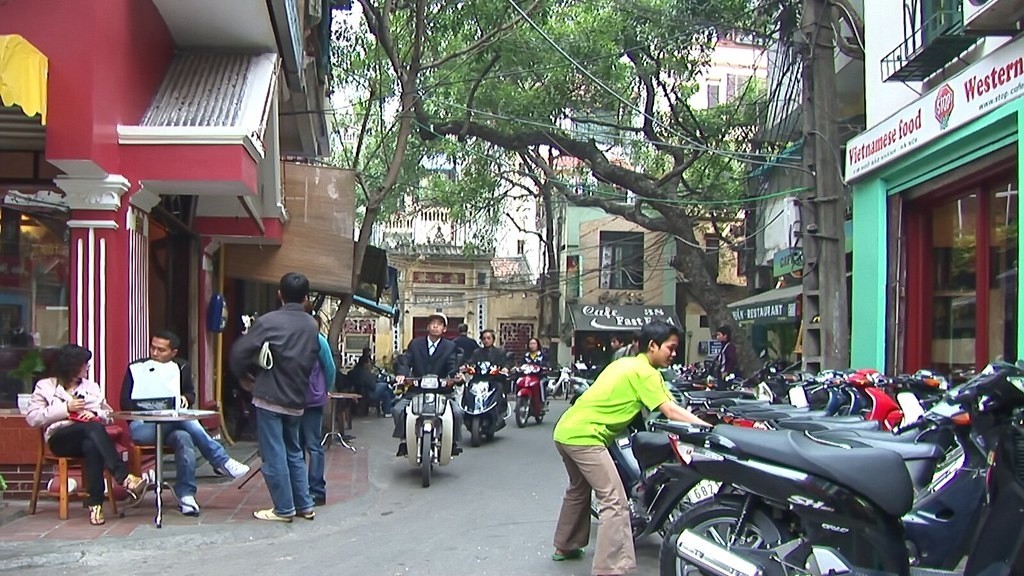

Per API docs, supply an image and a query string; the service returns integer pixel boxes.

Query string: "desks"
[321,392,362,453]
[109,409,221,528]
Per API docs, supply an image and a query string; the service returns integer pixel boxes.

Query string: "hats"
[428,311,447,328]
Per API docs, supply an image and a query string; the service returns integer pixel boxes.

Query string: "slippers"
[302,512,315,518]
[253,508,292,521]
[553,548,585,561]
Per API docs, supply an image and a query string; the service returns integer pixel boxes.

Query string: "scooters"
[457,349,515,447]
[512,363,552,427]
[546,365,572,401]
[569,350,1024,576]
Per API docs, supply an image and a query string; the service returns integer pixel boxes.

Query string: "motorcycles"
[394,375,459,488]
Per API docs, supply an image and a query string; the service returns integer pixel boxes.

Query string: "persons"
[392,312,467,457]
[349,324,639,434]
[25,344,148,525]
[229,272,321,522]
[552,320,713,576]
[120,330,250,515]
[717,325,736,391]
[298,300,335,505]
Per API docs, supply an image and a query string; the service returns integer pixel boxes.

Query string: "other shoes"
[88,504,104,525]
[385,413,392,417]
[396,443,407,456]
[452,442,462,452]
[123,474,146,500]
[310,494,325,506]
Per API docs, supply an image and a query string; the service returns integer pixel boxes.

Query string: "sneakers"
[178,495,199,515]
[216,460,250,479]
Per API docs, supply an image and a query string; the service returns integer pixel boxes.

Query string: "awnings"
[353,295,395,318]
[0,34,48,126]
[567,303,684,333]
[727,284,804,321]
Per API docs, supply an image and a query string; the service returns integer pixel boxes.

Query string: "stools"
[126,440,173,477]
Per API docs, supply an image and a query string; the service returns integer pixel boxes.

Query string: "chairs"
[29,427,118,519]
